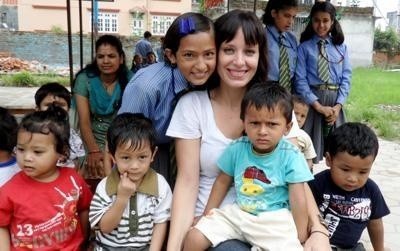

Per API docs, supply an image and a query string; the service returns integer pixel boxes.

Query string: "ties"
[317,39,329,82]
[279,35,292,91]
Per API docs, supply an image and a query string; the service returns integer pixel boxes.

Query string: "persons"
[0,0,391,251]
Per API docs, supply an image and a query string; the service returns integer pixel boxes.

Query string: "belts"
[311,83,339,90]
